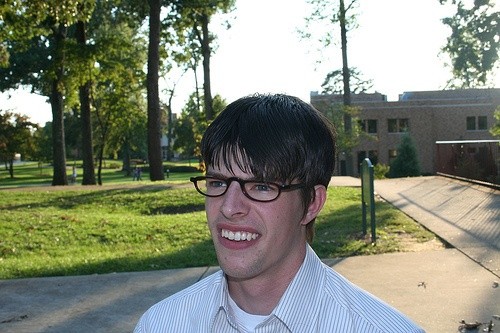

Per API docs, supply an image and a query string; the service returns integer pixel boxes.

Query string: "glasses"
[189,176,316,202]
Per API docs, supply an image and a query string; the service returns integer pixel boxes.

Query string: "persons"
[129,93,426,333]
